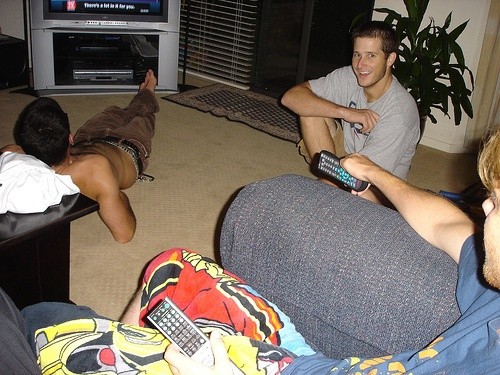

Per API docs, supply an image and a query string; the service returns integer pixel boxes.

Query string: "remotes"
[318,150,368,191]
[146,296,246,375]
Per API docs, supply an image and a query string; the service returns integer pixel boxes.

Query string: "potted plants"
[373,0,475,146]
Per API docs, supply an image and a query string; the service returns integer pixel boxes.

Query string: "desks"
[0,193,100,311]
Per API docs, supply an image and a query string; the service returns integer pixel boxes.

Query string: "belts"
[73,136,143,174]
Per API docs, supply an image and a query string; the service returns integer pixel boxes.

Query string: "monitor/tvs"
[30,0,181,33]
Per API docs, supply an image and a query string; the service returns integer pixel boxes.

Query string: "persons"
[0,70,159,244]
[280,20,421,208]
[19,131,500,375]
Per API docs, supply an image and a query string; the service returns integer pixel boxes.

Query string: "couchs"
[219,174,462,361]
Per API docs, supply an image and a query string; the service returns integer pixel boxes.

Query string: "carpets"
[161,80,303,144]
